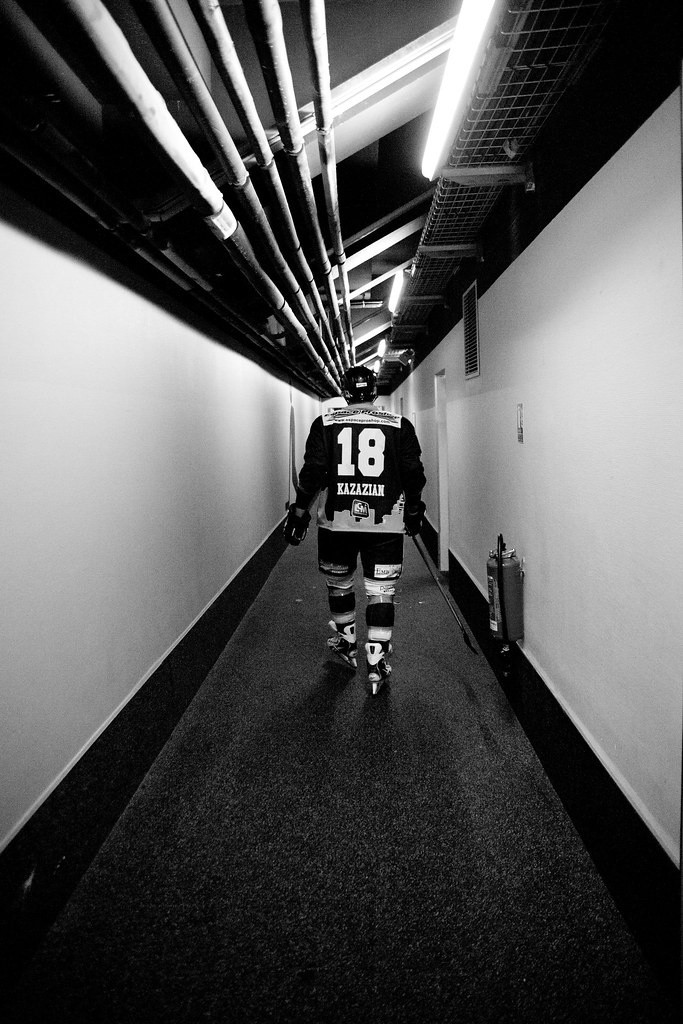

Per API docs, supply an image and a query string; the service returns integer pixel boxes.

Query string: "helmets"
[340,366,378,405]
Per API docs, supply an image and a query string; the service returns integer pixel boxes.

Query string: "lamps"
[386,268,409,313]
[422,0,498,182]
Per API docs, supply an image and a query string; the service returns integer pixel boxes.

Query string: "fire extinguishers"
[486,534,525,643]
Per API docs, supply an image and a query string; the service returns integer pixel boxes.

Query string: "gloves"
[403,500,427,537]
[283,503,312,546]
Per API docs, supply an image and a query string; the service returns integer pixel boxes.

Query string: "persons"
[283,365,427,695]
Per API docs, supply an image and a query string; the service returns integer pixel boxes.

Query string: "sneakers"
[364,642,394,698]
[326,620,359,670]
